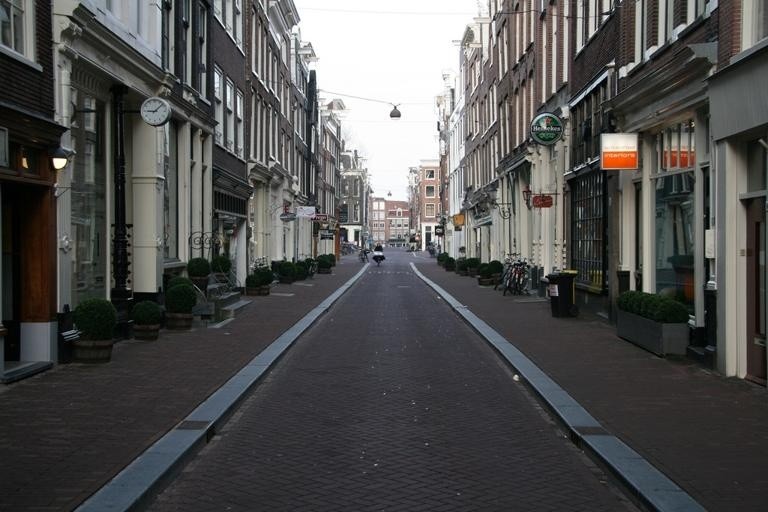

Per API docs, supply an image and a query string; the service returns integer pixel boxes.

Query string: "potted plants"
[615,289,690,358]
[436,251,504,287]
[71,253,333,366]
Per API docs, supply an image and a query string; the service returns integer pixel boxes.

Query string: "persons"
[373,243,385,260]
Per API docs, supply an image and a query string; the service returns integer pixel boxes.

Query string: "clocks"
[141,95,173,128]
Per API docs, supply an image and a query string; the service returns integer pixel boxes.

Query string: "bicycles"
[359,248,370,264]
[494,253,530,296]
[374,250,383,266]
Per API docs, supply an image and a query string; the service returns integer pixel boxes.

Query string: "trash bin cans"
[545,273,579,317]
